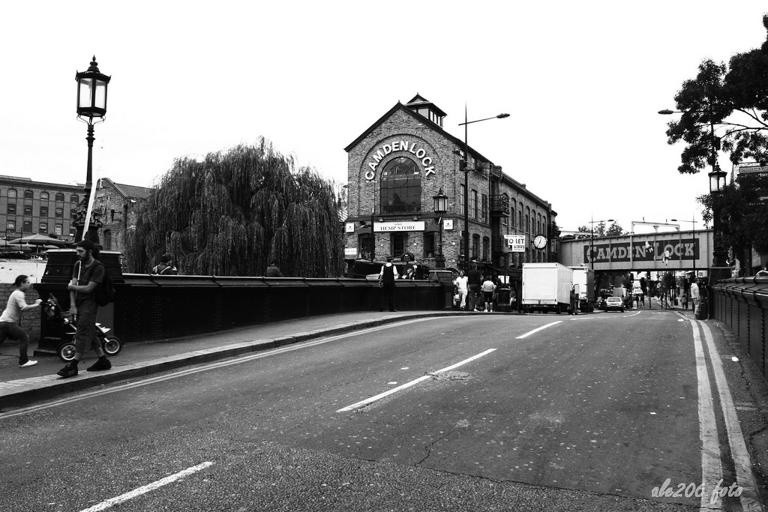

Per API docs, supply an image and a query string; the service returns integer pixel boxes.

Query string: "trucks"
[351,255,633,314]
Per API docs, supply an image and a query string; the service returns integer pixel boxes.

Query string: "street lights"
[669,217,699,278]
[432,187,449,270]
[71,52,112,242]
[656,105,716,174]
[588,216,615,270]
[706,160,729,268]
[456,102,510,268]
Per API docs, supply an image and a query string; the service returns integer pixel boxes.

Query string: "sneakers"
[19,360,38,368]
[87,359,110,370]
[57,364,78,377]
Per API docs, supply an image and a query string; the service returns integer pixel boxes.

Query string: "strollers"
[38,292,122,361]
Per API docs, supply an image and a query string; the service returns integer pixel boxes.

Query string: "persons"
[152,254,177,275]
[481,276,497,313]
[266,261,284,277]
[57,239,116,378]
[0,275,43,368]
[468,263,483,311]
[570,289,579,315]
[378,257,399,312]
[453,269,470,311]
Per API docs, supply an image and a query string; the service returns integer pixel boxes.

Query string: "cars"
[632,287,643,296]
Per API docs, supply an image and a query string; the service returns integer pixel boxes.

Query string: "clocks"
[533,235,548,249]
